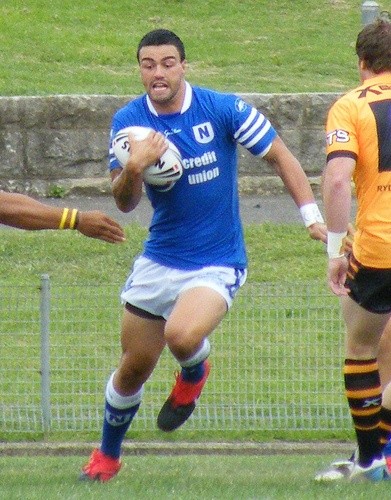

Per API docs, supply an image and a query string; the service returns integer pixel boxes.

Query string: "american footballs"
[112,125,183,185]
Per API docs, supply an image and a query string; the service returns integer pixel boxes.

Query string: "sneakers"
[382,438,391,480]
[313,448,387,483]
[77,447,123,483]
[157,358,210,432]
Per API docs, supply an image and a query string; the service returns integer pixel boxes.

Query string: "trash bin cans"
[362,1,379,24]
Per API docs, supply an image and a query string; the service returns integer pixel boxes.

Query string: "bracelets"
[326,230,349,258]
[299,203,324,228]
[59,208,78,230]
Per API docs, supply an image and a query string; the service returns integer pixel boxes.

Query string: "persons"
[0,190,126,244]
[321,20,391,486]
[76,29,328,481]
